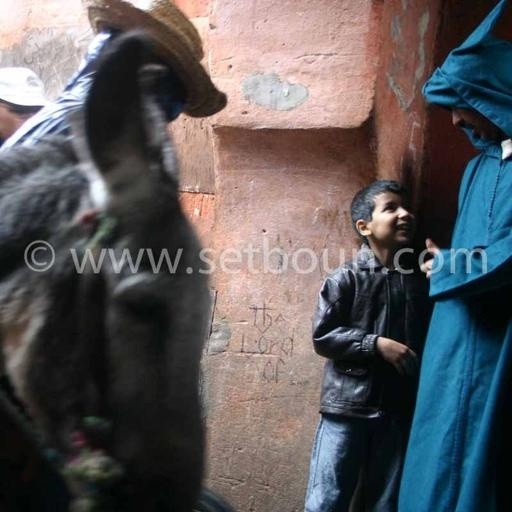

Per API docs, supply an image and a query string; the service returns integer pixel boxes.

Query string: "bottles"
[82,1,226,116]
[0,68,46,107]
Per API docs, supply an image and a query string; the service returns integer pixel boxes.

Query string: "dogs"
[0,29,213,512]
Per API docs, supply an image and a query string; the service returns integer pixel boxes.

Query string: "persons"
[0,67,50,145]
[392,1,511,511]
[304,179,429,510]
[0,0,229,176]
